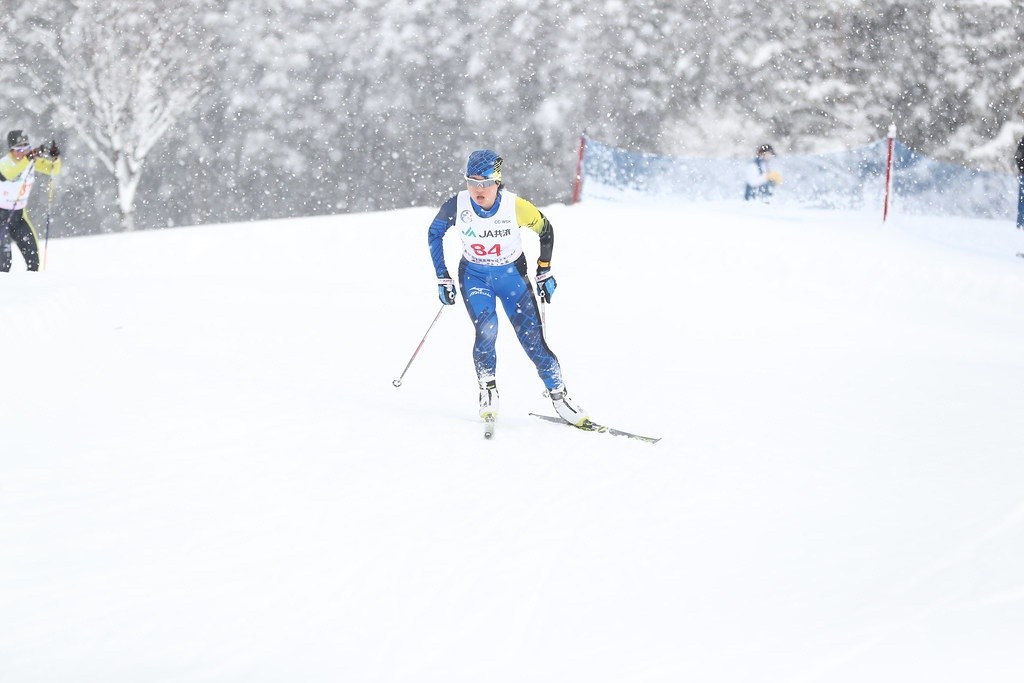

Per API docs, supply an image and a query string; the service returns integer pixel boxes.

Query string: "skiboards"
[483,411,664,444]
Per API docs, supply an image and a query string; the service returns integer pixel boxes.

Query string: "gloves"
[50,147,60,156]
[767,171,780,183]
[438,271,457,305]
[26,146,44,160]
[536,266,557,304]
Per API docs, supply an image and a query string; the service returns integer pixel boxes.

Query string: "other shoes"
[553,399,590,427]
[478,387,499,418]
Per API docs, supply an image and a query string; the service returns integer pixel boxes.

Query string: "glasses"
[11,146,31,153]
[464,175,496,188]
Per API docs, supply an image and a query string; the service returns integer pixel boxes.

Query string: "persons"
[0,129,61,272]
[427,150,583,426]
[744,144,781,205]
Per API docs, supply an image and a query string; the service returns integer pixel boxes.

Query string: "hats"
[466,150,503,181]
[8,129,29,151]
